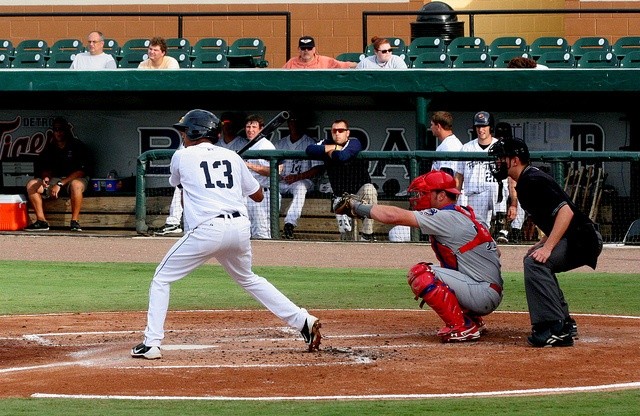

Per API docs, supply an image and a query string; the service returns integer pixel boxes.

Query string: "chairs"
[494,51,531,68]
[165,52,191,68]
[612,36,640,56]
[11,51,45,68]
[103,39,120,55]
[577,51,619,68]
[447,37,487,57]
[50,38,85,52]
[117,39,149,57]
[620,50,640,68]
[407,37,446,57]
[536,51,576,68]
[0,40,13,55]
[335,52,368,62]
[412,51,452,68]
[104,51,118,67]
[0,52,11,67]
[528,36,571,61]
[488,37,528,60]
[13,40,48,55]
[571,37,612,57]
[190,37,228,57]
[366,37,407,56]
[392,52,412,68]
[452,52,493,68]
[226,38,263,67]
[118,52,143,68]
[165,38,191,54]
[46,52,77,68]
[192,52,228,68]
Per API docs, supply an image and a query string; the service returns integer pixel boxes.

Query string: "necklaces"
[375,56,389,68]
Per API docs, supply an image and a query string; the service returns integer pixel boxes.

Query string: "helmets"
[406,169,463,211]
[488,137,528,181]
[170,109,222,144]
[473,110,495,134]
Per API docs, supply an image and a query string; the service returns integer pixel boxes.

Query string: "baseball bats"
[237,111,289,156]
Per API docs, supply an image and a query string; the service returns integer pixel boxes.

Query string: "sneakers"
[153,223,183,236]
[493,229,510,243]
[441,322,481,343]
[473,315,488,334]
[360,234,371,243]
[131,342,162,359]
[526,328,574,348]
[532,319,578,337]
[22,220,50,232]
[284,223,294,238]
[70,221,82,232]
[300,315,322,352]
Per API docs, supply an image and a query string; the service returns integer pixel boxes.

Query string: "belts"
[218,212,240,218]
[264,188,270,192]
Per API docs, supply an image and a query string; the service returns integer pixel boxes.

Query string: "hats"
[299,36,314,47]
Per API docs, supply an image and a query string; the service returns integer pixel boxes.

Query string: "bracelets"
[334,144,339,152]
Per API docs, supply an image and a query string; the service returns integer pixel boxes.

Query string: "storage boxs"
[0,194,29,231]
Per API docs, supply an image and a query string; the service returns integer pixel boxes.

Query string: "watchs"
[58,181,62,185]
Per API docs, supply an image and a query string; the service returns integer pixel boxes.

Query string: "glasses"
[300,46,314,50]
[378,49,392,53]
[333,128,349,134]
[89,40,102,44]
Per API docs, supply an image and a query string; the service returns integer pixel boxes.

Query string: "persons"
[355,35,408,70]
[214,112,249,152]
[428,111,464,197]
[488,137,604,347]
[275,111,326,238]
[153,142,184,236]
[281,36,358,69]
[235,115,283,240]
[330,169,504,342]
[21,117,91,232]
[68,30,118,70]
[506,56,548,67]
[305,119,378,242]
[456,111,525,243]
[129,107,322,361]
[136,35,180,69]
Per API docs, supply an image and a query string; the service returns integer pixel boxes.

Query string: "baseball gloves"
[334,192,368,218]
